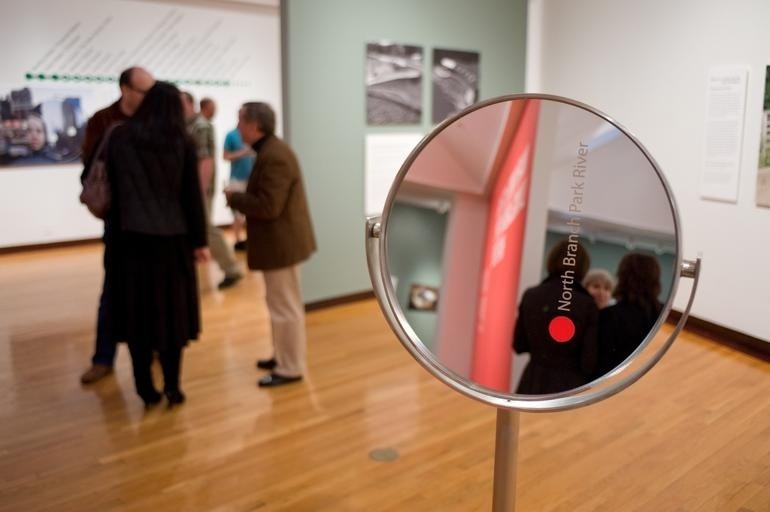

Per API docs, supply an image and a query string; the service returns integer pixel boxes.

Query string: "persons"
[81,82,211,406]
[581,268,617,308]
[597,254,664,377]
[225,102,317,386]
[27,113,51,156]
[178,93,257,290]
[80,70,154,387]
[513,242,599,395]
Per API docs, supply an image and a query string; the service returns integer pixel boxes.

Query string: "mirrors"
[361,92,704,418]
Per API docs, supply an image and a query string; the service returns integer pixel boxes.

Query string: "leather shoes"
[257,372,303,387]
[234,241,248,251]
[79,362,115,384]
[256,357,277,369]
[218,273,245,289]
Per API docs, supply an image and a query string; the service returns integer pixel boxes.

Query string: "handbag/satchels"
[78,122,122,219]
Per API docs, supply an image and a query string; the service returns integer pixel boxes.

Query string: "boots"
[126,337,162,408]
[158,341,185,407]
